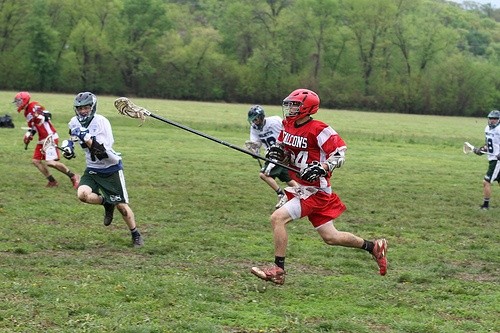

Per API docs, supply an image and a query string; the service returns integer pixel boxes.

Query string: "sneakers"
[275,194,288,208]
[372,238,387,276]
[103,204,115,225]
[132,233,142,248]
[71,174,81,189]
[252,266,286,285]
[47,181,59,187]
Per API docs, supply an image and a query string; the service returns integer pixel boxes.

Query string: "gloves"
[24,132,34,144]
[28,115,44,129]
[266,146,284,162]
[300,160,326,182]
[62,146,74,160]
[473,146,487,156]
[69,126,89,140]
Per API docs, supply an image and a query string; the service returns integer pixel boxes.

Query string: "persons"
[12,91,81,190]
[251,88,388,285]
[61,92,143,249]
[473,110,500,210]
[244,104,299,209]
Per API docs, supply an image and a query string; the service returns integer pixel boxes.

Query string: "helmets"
[73,91,96,125]
[487,110,500,128]
[282,89,320,127]
[247,106,266,131]
[13,92,30,113]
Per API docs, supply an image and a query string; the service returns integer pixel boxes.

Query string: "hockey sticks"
[42,135,77,159]
[463,141,497,156]
[24,105,40,150]
[114,97,302,174]
[245,142,262,169]
